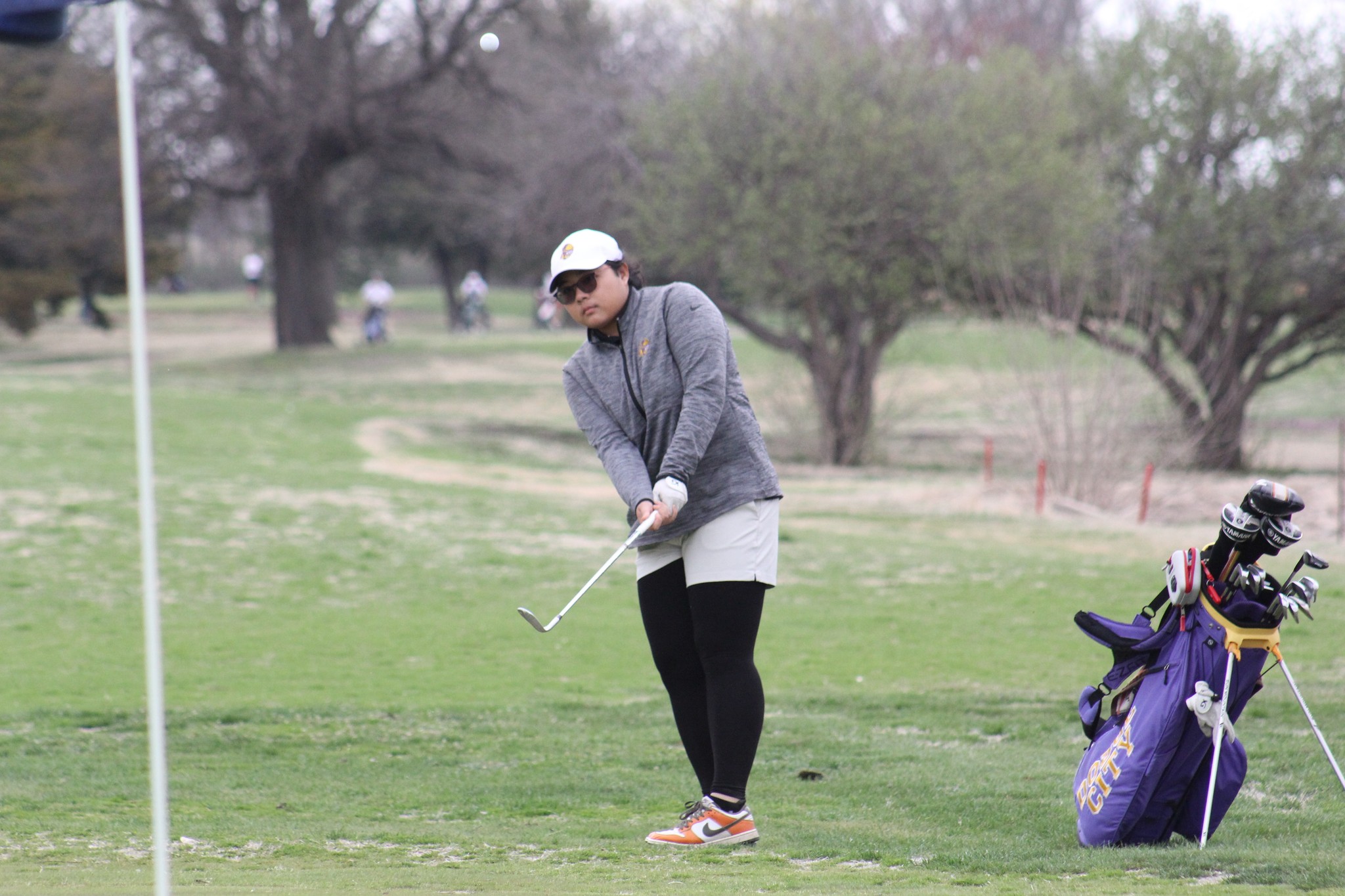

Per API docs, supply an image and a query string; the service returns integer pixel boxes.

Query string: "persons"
[538,229,787,846]
[242,244,265,300]
[460,270,488,334]
[360,269,392,343]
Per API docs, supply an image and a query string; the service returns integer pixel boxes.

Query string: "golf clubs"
[1217,549,1332,625]
[516,508,660,633]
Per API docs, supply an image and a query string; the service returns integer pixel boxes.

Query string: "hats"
[548,228,623,293]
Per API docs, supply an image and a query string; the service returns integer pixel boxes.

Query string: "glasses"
[554,264,616,305]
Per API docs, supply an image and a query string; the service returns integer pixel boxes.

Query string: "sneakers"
[647,794,760,850]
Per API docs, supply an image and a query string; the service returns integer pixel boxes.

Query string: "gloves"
[652,475,689,516]
